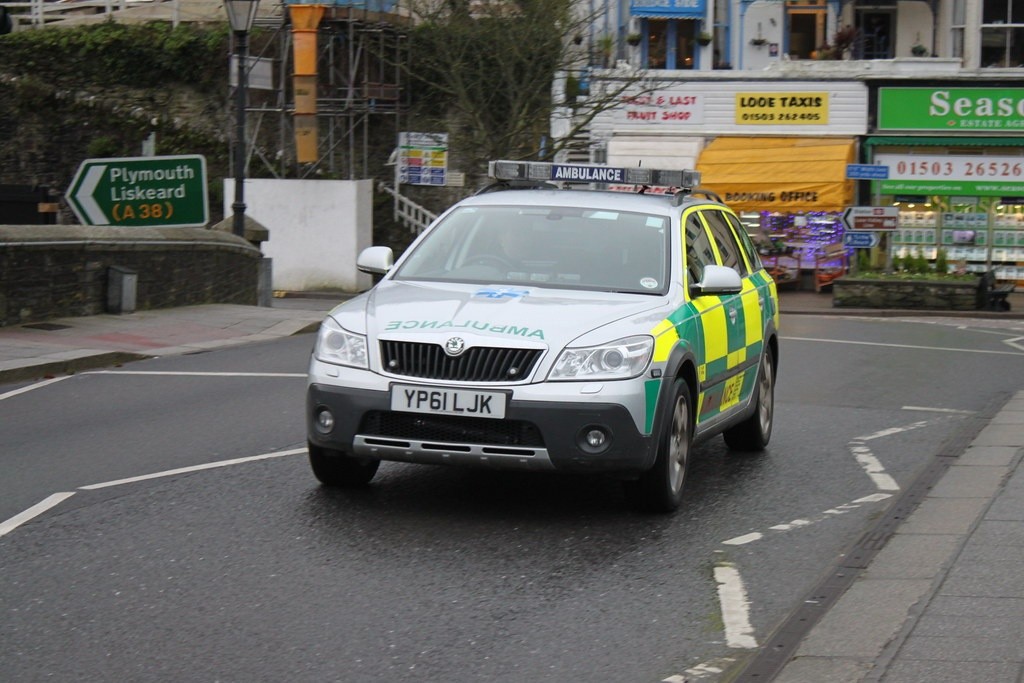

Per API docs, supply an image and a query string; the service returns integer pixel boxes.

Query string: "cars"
[303,160,780,514]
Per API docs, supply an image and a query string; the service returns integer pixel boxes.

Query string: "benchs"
[981,270,1016,312]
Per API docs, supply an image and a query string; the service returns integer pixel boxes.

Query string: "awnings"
[628,0,707,22]
[690,136,856,218]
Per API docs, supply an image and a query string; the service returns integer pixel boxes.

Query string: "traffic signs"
[840,207,899,232]
[842,231,882,249]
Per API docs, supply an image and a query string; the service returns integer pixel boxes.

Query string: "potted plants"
[623,32,644,46]
[688,32,713,46]
[911,44,927,57]
[825,24,871,60]
